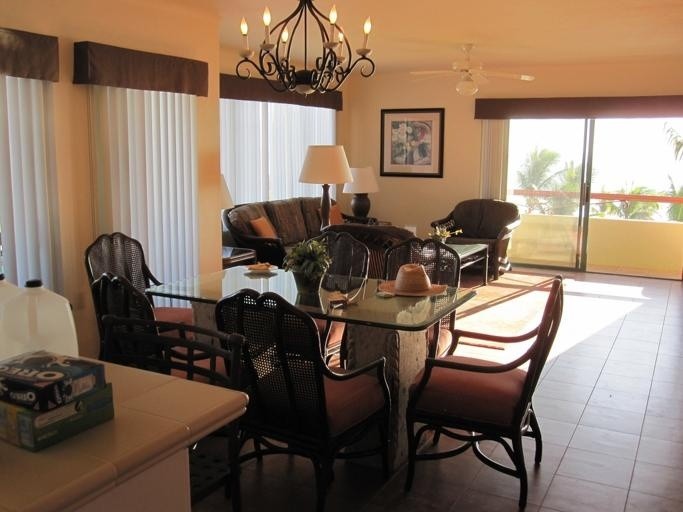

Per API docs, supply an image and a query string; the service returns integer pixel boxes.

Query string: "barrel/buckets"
[1,279,80,361]
[0,265,18,290]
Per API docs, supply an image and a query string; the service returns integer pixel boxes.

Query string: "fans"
[409,44,535,85]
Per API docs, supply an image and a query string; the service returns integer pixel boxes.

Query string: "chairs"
[91,272,162,370]
[431,199,520,275]
[99,315,243,512]
[321,224,415,281]
[215,289,393,512]
[340,238,461,370]
[83,232,186,369]
[404,276,563,512]
[300,232,371,365]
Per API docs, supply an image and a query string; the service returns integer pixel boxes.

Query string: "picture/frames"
[380,108,444,178]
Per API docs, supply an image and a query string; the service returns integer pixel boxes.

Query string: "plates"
[244,265,279,274]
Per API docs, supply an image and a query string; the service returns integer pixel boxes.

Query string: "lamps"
[342,168,380,217]
[298,145,354,228]
[220,174,235,209]
[236,0,376,98]
[455,77,478,96]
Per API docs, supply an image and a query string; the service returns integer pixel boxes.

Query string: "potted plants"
[419,227,464,244]
[282,238,333,295]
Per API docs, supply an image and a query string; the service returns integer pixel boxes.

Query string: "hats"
[378,263,447,296]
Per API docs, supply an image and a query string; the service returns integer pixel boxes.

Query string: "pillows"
[317,204,345,225]
[250,216,278,239]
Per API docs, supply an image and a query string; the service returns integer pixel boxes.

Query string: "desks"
[222,246,256,264]
[145,264,475,478]
[0,355,250,512]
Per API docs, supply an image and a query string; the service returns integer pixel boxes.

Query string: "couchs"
[223,197,367,269]
